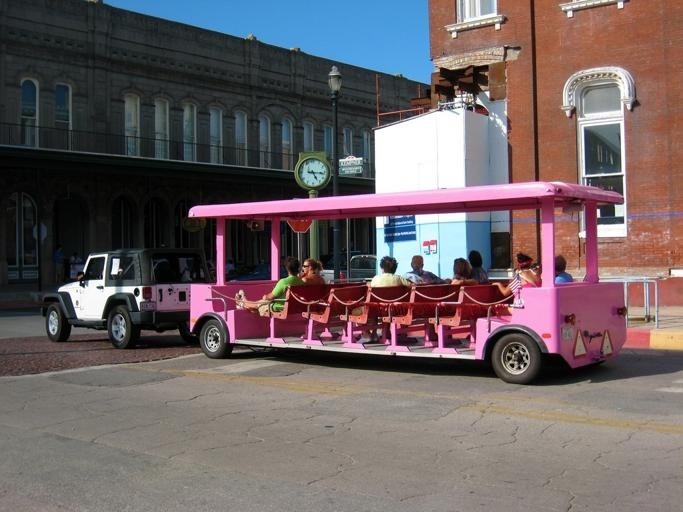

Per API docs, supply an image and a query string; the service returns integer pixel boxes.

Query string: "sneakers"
[356,335,372,343]
[239,289,247,302]
[235,292,247,311]
[368,333,378,342]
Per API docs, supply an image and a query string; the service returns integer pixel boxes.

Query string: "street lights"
[327,63,343,282]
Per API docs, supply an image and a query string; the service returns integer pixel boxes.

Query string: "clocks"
[294,151,330,190]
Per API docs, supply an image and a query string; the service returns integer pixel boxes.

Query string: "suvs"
[39,247,211,349]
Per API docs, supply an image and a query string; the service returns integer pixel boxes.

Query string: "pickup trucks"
[320,253,376,283]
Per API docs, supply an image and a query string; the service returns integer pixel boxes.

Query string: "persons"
[299,258,326,284]
[70,251,83,263]
[555,255,574,284]
[402,255,450,286]
[235,257,305,317]
[351,256,416,343]
[54,245,65,284]
[450,258,480,285]
[492,253,542,315]
[469,251,489,285]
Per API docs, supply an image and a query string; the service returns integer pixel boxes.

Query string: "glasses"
[302,265,309,268]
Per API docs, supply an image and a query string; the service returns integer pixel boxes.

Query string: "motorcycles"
[187,181,627,385]
